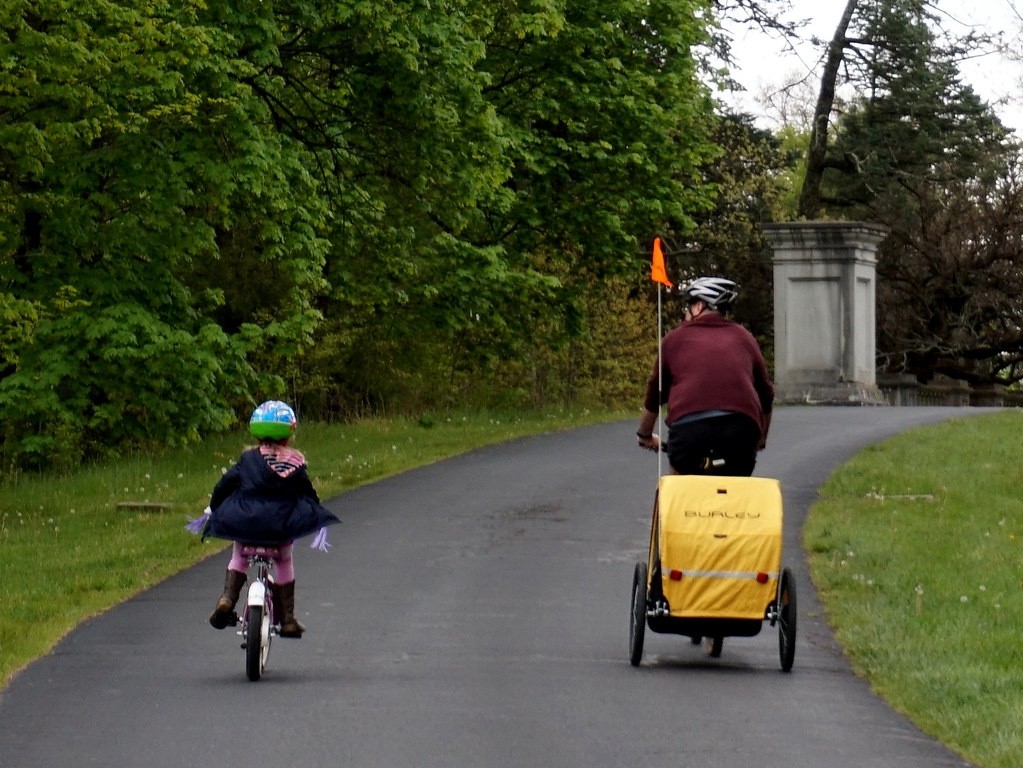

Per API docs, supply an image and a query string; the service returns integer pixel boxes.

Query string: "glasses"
[682,304,696,312]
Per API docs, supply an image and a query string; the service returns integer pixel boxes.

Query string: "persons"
[202,401,340,636]
[636,277,774,477]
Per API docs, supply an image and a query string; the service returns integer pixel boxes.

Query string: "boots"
[210,568,248,630]
[275,579,306,637]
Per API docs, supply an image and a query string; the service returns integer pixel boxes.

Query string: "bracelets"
[636,428,652,439]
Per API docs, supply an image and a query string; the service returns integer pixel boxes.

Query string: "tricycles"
[628,440,798,672]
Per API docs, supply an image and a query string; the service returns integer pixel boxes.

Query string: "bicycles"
[229,546,303,681]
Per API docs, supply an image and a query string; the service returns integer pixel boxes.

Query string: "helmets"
[685,277,738,310]
[249,400,297,439]
